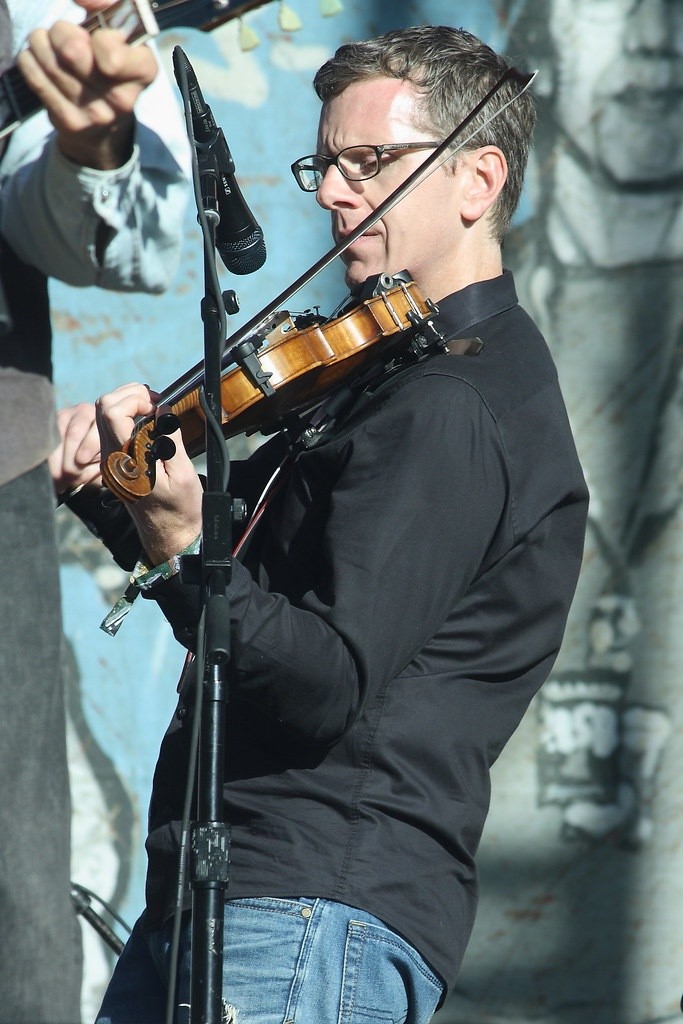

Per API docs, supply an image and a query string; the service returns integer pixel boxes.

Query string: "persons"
[0,0,192,1024]
[47,21,592,1024]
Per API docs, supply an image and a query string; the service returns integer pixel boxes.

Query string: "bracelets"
[100,532,201,637]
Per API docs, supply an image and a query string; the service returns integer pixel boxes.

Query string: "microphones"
[173,48,267,275]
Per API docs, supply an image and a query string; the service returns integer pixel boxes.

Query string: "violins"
[98,269,437,506]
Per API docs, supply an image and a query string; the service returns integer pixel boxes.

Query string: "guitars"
[0,0,280,141]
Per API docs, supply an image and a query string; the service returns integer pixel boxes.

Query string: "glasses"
[289,138,470,193]
